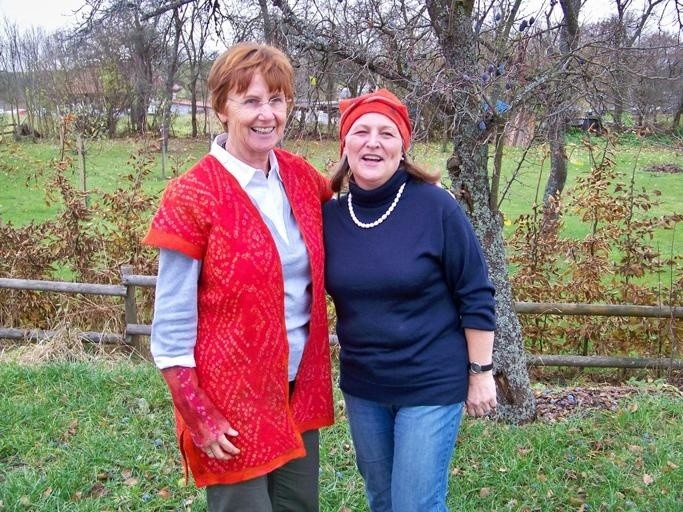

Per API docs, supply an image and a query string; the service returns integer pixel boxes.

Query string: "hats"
[339,88,412,158]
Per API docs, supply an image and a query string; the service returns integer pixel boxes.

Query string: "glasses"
[229,96,286,111]
